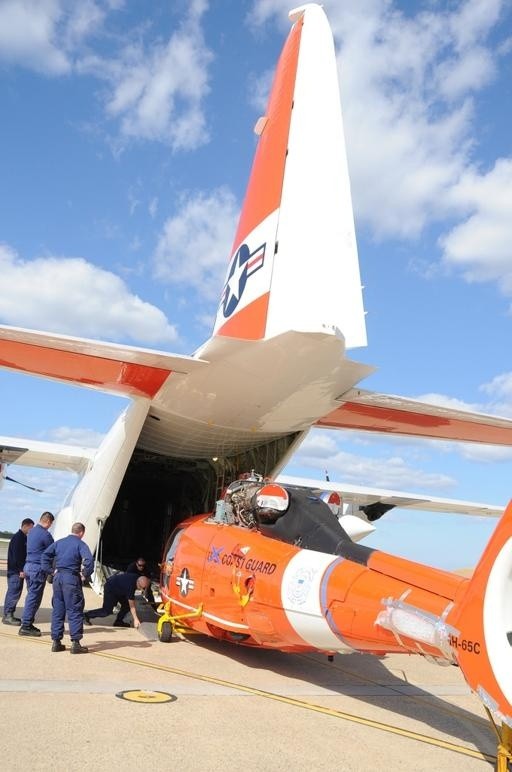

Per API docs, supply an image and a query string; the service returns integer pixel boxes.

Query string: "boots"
[1,610,130,654]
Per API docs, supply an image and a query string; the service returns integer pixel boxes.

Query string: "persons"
[40,522,94,653]
[17,512,55,636]
[126,557,157,610]
[83,573,150,626]
[2,517,34,627]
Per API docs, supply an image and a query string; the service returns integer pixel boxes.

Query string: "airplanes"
[0,6,511,609]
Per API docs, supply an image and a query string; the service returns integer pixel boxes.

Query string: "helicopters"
[152,470,512,729]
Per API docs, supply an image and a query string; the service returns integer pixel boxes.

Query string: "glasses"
[136,563,147,567]
